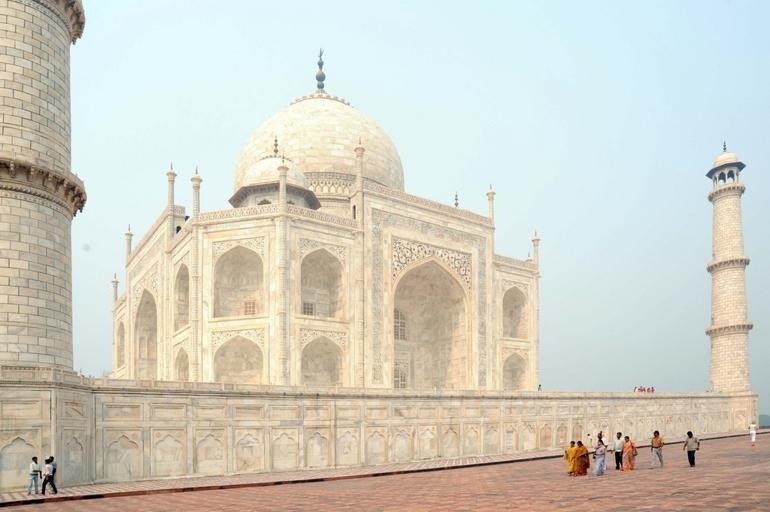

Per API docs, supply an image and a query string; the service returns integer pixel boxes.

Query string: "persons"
[682,430,700,467]
[747,419,759,447]
[620,435,638,472]
[597,433,607,470]
[27,456,43,495]
[593,438,608,476]
[648,430,665,470]
[563,441,576,475]
[43,456,56,494]
[569,440,590,475]
[610,431,623,470]
[40,458,57,494]
